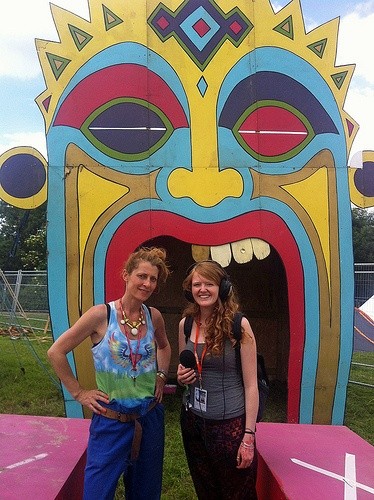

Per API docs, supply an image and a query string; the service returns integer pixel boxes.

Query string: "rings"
[237,456,240,460]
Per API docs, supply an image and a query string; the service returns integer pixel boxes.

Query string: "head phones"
[184,260,233,303]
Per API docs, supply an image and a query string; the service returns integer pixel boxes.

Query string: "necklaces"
[120,301,145,336]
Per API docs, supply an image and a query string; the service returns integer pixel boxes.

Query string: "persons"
[48,245,171,500]
[177,260,261,500]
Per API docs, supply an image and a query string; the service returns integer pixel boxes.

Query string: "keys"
[133,377,137,387]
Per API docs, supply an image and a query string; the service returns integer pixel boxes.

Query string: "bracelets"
[241,428,255,450]
[157,369,168,384]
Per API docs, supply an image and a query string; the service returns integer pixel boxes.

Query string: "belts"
[94,398,157,461]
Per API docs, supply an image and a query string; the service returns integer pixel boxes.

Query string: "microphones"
[179,349,196,388]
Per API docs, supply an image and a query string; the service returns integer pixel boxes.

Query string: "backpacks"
[183,312,269,423]
